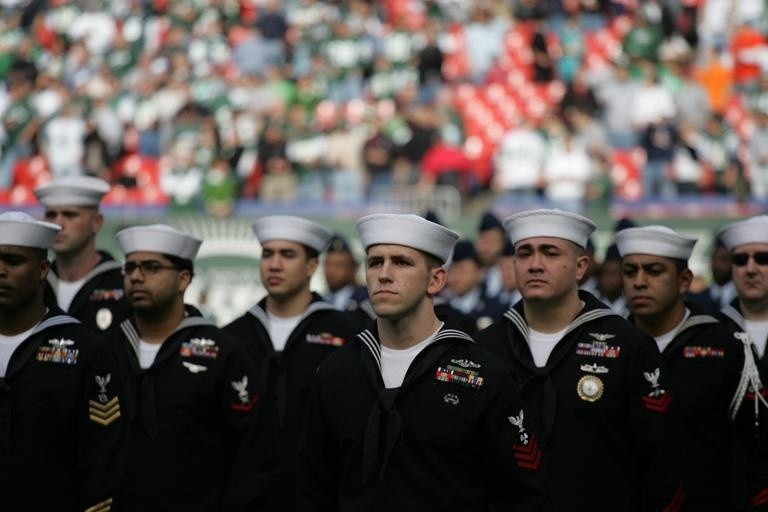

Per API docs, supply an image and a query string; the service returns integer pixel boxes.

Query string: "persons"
[1,1,767,336]
[611,224,766,512]
[97,224,267,512]
[469,208,673,512]
[307,214,556,512]
[712,214,766,511]
[31,177,131,356]
[0,209,133,512]
[220,214,361,512]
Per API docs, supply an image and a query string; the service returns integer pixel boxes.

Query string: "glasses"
[124,260,176,275]
[731,253,768,265]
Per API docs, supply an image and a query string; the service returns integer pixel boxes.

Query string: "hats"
[356,213,459,264]
[614,224,698,259]
[328,232,348,250]
[505,208,596,247]
[253,215,333,253]
[114,224,202,261]
[0,212,63,250]
[718,215,768,251]
[32,177,110,208]
[453,212,514,263]
